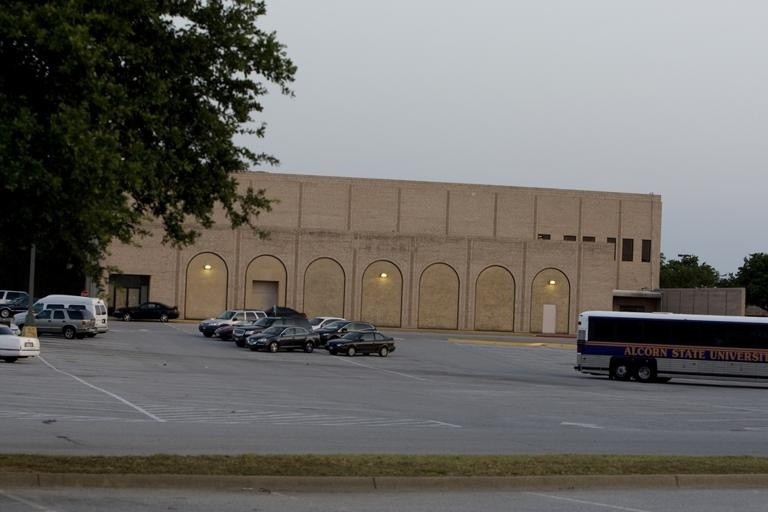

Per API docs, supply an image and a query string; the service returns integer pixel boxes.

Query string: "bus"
[573,311,768,389]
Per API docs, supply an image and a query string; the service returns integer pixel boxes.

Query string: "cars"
[0,290,99,363]
[199,304,396,357]
[112,301,179,323]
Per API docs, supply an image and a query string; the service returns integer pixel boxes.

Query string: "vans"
[13,294,109,337]
